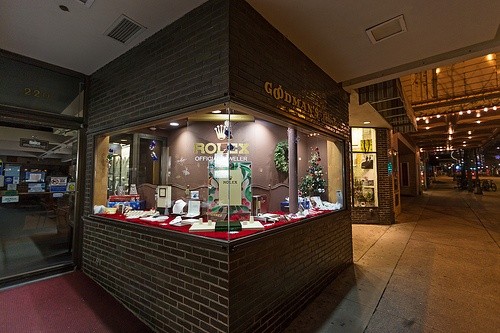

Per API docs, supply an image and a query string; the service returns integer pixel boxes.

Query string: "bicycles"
[482,179,497,192]
